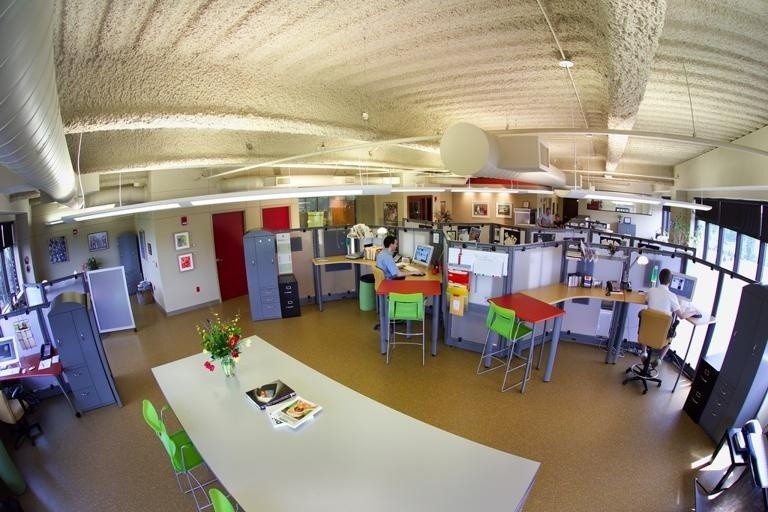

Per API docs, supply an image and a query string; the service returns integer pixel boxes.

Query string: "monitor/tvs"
[412,244,435,267]
[0,337,20,369]
[669,271,698,302]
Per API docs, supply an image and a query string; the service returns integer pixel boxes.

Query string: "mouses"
[22,368,27,374]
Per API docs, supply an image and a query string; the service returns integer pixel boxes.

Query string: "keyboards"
[404,265,419,271]
[0,366,21,377]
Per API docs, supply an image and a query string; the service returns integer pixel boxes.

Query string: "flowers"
[196,307,244,372]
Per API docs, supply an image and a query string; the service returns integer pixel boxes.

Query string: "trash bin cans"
[359,274,376,312]
[136,280,153,305]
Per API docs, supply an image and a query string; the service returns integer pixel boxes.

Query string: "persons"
[637,269,702,368]
[535,207,553,228]
[552,213,564,229]
[376,234,425,282]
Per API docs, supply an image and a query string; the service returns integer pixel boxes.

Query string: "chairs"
[0,390,43,450]
[142,399,232,512]
[476,304,533,393]
[386,292,426,366]
[208,488,239,512]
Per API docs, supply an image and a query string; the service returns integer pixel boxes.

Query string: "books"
[265,401,313,428]
[244,379,296,411]
[364,246,383,261]
[270,396,323,429]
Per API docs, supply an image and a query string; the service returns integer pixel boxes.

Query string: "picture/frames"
[495,203,513,219]
[175,231,190,251]
[472,203,490,218]
[87,231,109,251]
[179,253,194,271]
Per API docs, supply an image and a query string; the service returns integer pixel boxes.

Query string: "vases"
[221,356,235,376]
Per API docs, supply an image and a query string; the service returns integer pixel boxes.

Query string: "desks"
[0,349,81,418]
[710,428,749,494]
[312,255,443,338]
[150,335,542,512]
[521,282,717,392]
[376,280,441,357]
[487,292,566,393]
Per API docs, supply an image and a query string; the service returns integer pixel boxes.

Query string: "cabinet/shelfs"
[278,274,301,319]
[243,228,282,322]
[48,292,116,414]
[118,236,142,295]
[699,284,767,446]
[684,352,725,424]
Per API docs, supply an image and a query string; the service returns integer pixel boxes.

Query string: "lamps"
[61,184,391,225]
[570,189,711,211]
[625,255,649,291]
[393,189,556,194]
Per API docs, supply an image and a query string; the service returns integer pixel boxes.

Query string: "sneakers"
[641,352,662,368]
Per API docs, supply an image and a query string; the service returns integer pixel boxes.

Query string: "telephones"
[607,280,621,292]
